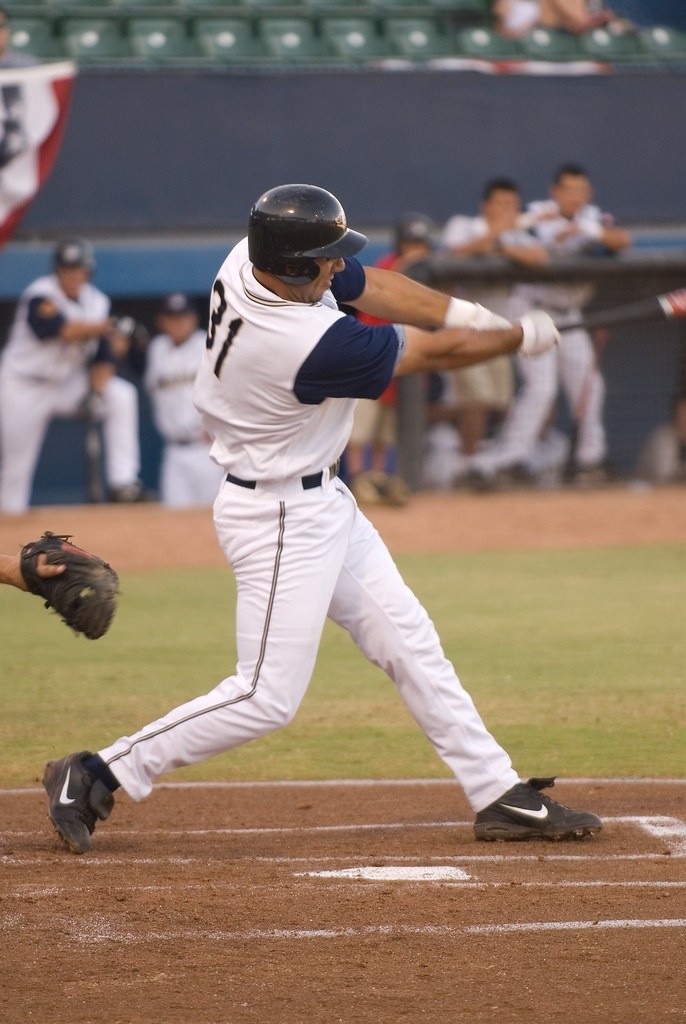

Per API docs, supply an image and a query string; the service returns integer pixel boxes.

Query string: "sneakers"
[473,776,603,842]
[42,751,115,854]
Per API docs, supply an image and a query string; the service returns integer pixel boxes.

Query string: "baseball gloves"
[21,531,123,642]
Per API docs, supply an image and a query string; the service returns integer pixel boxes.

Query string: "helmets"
[160,294,195,315]
[248,184,368,285]
[57,236,95,270]
[395,212,441,250]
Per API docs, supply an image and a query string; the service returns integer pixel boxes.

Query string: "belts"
[226,459,341,489]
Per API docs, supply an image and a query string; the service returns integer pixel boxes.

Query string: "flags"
[0,58,78,250]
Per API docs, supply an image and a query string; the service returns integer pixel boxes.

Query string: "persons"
[335,161,639,494]
[143,293,227,506]
[0,237,152,515]
[0,531,120,639]
[44,184,603,856]
[490,0,619,38]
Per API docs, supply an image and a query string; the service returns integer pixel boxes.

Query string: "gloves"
[520,311,560,359]
[445,296,513,334]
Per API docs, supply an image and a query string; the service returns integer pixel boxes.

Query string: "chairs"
[0,1,686,72]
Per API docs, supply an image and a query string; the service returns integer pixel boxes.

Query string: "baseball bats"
[556,288,686,332]
